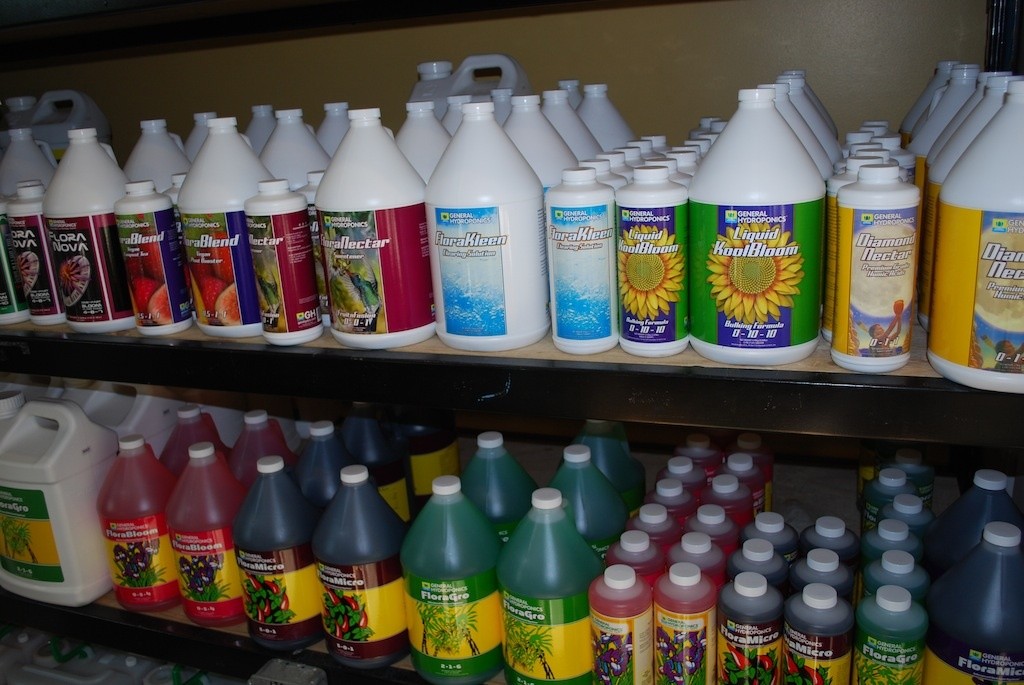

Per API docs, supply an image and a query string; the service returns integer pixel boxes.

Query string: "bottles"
[2,380,1022,684]
[0,51,1023,398]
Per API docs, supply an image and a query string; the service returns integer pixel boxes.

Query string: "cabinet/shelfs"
[0,0,1023,685]
